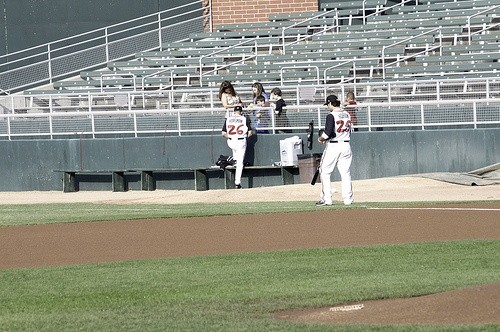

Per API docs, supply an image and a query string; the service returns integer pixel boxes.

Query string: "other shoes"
[315,200,332,206]
[235,185,242,189]
[344,199,354,205]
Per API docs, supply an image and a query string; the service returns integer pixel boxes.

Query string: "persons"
[343,90,358,131]
[270,87,292,134]
[222,105,252,189]
[315,94,354,206]
[252,95,270,134]
[218,80,251,123]
[246,82,270,134]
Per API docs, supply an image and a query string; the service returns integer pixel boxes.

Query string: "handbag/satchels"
[216,154,235,170]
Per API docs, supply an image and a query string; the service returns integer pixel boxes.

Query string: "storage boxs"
[279,139,303,166]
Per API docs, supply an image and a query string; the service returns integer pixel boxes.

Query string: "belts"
[229,138,244,140]
[330,140,349,143]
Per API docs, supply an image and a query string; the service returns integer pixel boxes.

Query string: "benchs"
[23,0,500,105]
[53,165,299,193]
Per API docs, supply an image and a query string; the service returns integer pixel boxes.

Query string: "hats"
[234,106,243,111]
[324,95,340,106]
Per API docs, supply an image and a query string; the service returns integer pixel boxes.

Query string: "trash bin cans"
[296,153,323,184]
